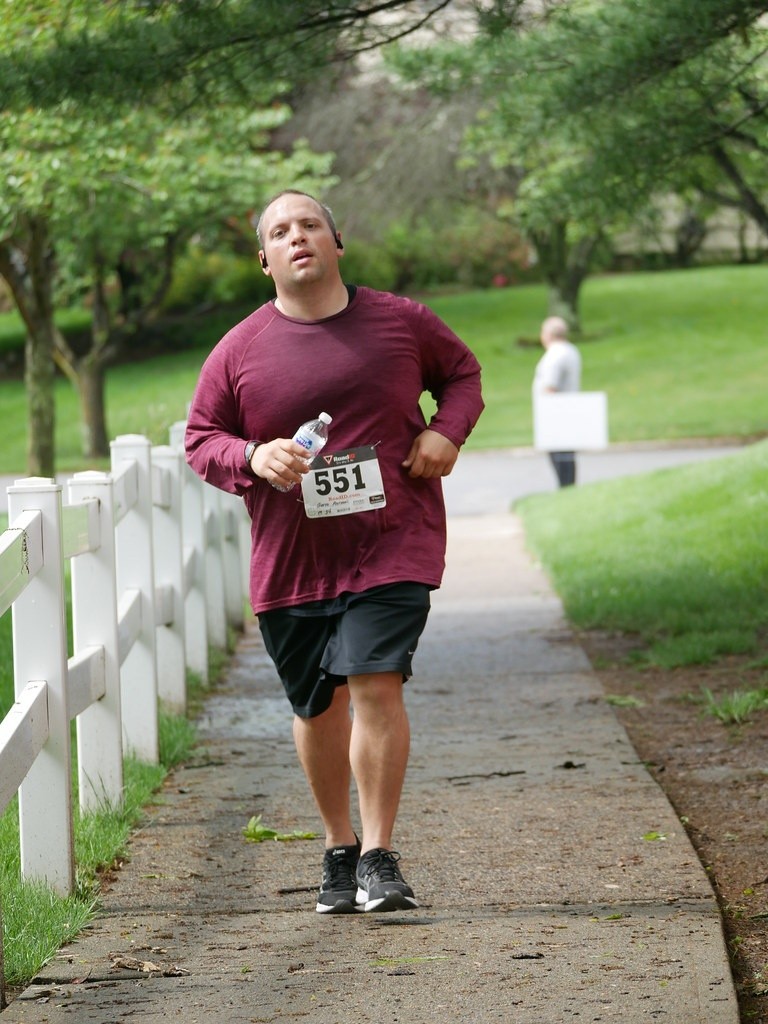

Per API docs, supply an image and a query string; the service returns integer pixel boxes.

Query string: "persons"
[531,315,583,490]
[183,188,485,914]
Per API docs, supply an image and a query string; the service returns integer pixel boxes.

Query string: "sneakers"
[355,847,417,910]
[315,832,362,914]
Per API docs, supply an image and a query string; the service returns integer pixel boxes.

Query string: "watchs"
[244,440,262,464]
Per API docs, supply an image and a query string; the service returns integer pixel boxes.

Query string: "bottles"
[267,411,333,493]
